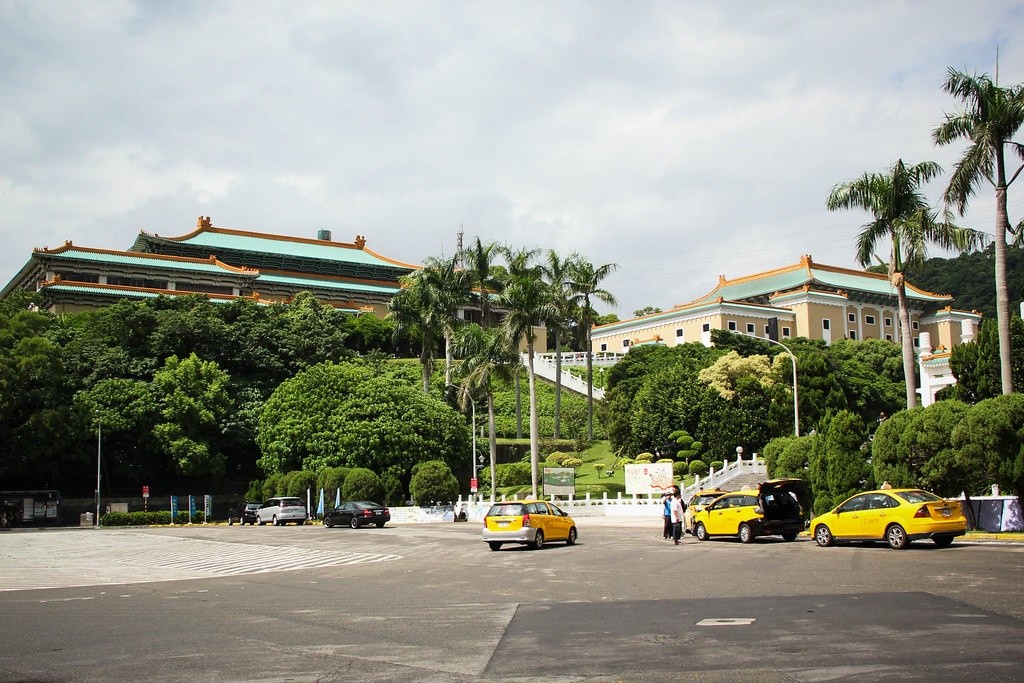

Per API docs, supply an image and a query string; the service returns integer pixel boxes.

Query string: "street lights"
[731,328,800,440]
[443,380,477,495]
[598,367,604,392]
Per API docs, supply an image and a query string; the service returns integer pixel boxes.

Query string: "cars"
[810,481,967,549]
[323,501,390,529]
[256,496,308,526]
[228,502,263,525]
[481,500,577,549]
[690,477,808,544]
[682,489,730,536]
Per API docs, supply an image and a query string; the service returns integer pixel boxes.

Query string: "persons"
[700,487,704,492]
[756,482,762,490]
[661,485,686,545]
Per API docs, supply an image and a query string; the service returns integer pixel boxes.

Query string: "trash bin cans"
[80,513,94,526]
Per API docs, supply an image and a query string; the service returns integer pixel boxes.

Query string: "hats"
[667,492,671,496]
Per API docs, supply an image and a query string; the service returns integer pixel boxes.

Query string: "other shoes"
[674,541,683,546]
